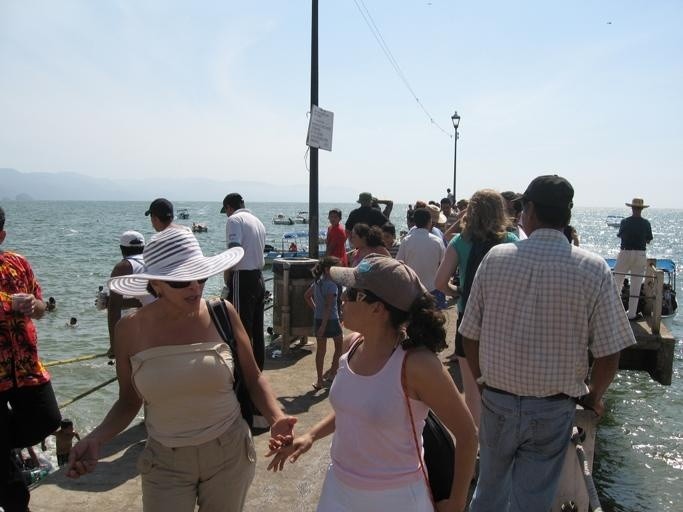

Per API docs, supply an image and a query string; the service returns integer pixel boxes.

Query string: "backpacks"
[205,296,274,439]
[343,334,479,511]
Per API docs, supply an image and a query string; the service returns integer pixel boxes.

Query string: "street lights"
[451,107,462,205]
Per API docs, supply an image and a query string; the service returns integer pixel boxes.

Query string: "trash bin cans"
[272,257,322,354]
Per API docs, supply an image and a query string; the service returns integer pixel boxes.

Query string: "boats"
[597,251,679,333]
[605,214,625,227]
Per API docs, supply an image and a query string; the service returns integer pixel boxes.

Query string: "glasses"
[160,277,208,288]
[346,288,376,304]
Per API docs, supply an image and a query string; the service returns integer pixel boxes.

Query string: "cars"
[165,202,353,269]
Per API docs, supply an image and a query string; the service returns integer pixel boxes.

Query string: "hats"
[145,198,173,218]
[356,192,372,202]
[108,225,244,298]
[220,193,244,213]
[329,252,427,312]
[425,204,448,224]
[120,231,145,247]
[625,198,650,208]
[510,175,576,208]
[329,208,341,218]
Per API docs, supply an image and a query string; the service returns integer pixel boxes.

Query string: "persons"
[45,297,56,311]
[38,417,82,465]
[612,197,653,324]
[0,203,61,511]
[64,317,78,327]
[12,446,39,467]
[455,176,636,511]
[95,175,593,443]
[264,251,479,510]
[63,226,299,512]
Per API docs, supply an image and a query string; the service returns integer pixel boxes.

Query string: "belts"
[479,384,579,402]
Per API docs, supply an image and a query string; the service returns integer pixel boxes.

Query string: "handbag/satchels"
[0,379,62,451]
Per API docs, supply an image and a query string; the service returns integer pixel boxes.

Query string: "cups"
[12,293,31,312]
